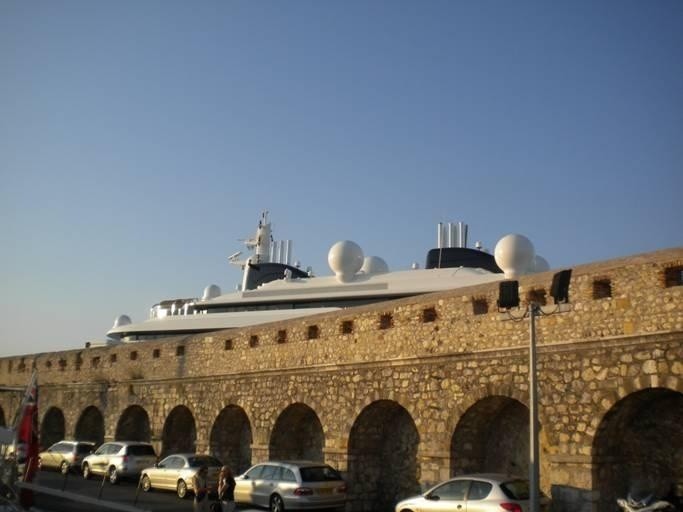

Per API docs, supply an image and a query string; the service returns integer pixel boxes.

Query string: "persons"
[216,465,236,511]
[192,464,214,512]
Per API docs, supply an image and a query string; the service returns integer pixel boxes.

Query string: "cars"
[3,442,33,460]
[79,441,159,485]
[139,452,226,499]
[37,440,97,475]
[233,461,345,511]
[395,472,552,511]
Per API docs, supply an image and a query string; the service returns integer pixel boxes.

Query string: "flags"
[14,373,40,484]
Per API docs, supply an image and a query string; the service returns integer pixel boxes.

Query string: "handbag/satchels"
[217,477,230,499]
[197,491,207,503]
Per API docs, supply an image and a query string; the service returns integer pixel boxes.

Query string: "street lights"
[496,269,571,510]
